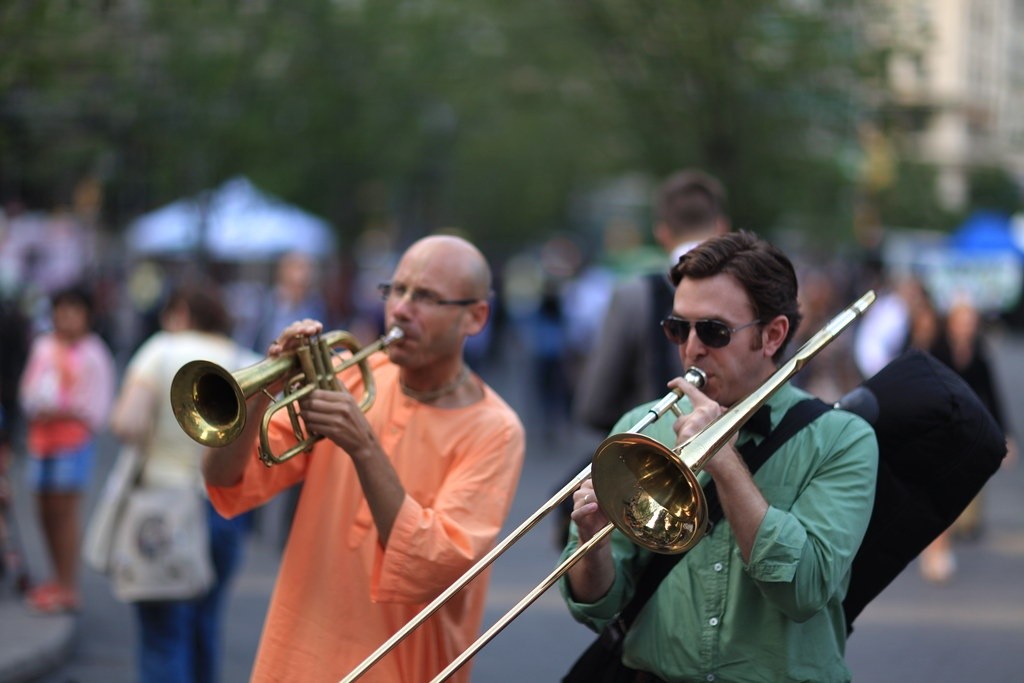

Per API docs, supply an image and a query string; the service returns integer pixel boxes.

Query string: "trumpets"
[168,326,404,468]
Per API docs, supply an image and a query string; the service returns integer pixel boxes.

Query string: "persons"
[462,205,676,459]
[108,288,274,682]
[17,285,121,617]
[0,164,394,558]
[201,235,528,683]
[553,231,879,683]
[787,225,1019,583]
[575,169,737,438]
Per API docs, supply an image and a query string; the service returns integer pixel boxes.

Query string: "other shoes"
[920,550,954,579]
[25,593,80,615]
[31,582,57,595]
[951,504,985,539]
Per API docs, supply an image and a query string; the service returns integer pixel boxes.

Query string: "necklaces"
[400,366,469,400]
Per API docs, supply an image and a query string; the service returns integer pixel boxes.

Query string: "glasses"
[378,283,478,307]
[660,316,762,348]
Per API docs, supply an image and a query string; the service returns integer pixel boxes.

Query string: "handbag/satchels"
[560,348,1008,683]
[83,446,143,574]
[108,485,214,602]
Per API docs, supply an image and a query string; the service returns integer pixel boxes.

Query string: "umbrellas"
[119,167,348,266]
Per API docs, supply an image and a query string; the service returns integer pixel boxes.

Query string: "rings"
[584,494,591,504]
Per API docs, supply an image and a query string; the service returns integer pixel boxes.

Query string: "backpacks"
[646,273,684,397]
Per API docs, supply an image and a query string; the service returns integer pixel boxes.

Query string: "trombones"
[338,289,877,683]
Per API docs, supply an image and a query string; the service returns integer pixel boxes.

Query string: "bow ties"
[740,405,772,436]
